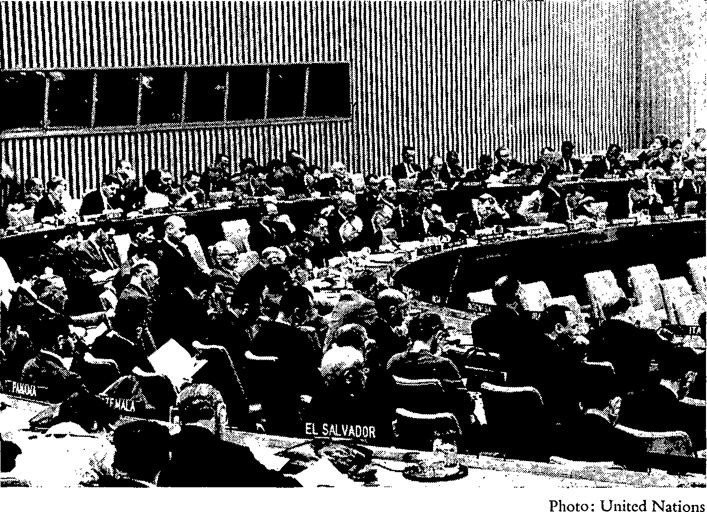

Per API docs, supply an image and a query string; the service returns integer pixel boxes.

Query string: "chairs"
[0,149,704,487]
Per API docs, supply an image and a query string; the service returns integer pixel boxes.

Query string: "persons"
[1,126,704,486]
